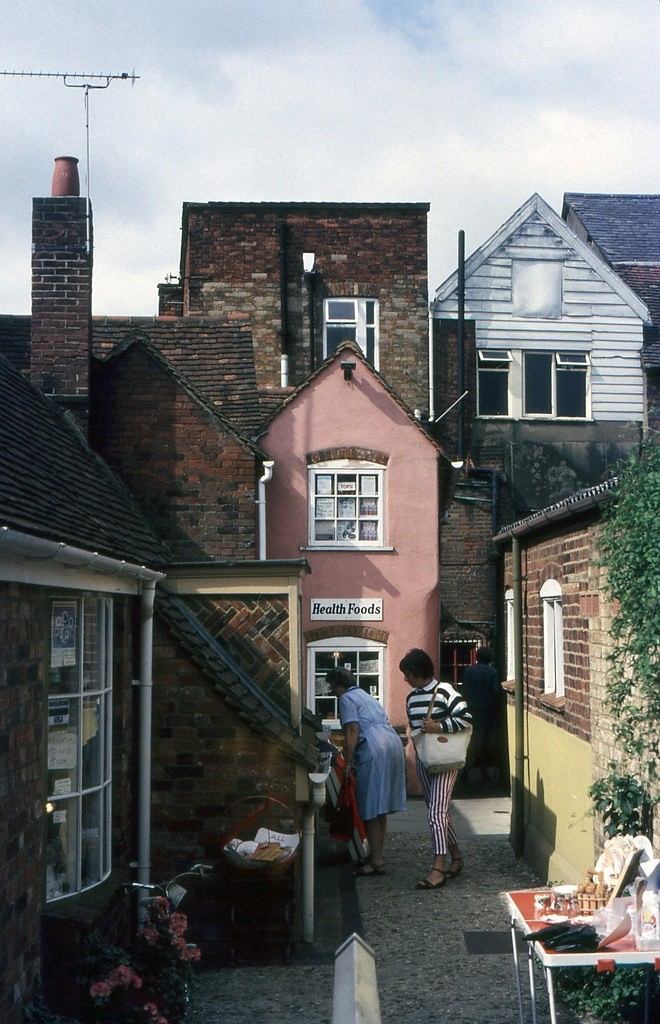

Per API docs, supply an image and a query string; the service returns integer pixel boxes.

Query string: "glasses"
[404,672,413,676]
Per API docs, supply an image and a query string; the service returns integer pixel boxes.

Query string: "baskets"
[216,795,303,881]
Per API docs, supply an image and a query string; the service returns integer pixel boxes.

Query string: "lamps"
[341,361,356,381]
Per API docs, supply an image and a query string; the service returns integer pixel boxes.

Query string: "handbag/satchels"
[409,682,472,772]
[328,777,355,837]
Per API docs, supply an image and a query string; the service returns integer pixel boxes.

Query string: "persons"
[462,647,506,782]
[325,667,404,877]
[399,648,475,890]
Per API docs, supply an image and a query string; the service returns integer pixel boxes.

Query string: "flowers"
[24,896,202,1024]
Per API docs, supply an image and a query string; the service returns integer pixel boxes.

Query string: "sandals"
[445,857,465,878]
[416,868,446,888]
[353,855,385,876]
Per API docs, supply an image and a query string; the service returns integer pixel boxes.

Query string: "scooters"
[117,863,215,1024]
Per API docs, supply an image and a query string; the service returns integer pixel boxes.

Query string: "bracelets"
[440,724,443,731]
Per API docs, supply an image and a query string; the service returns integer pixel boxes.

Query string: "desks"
[223,858,302,967]
[503,886,660,1024]
